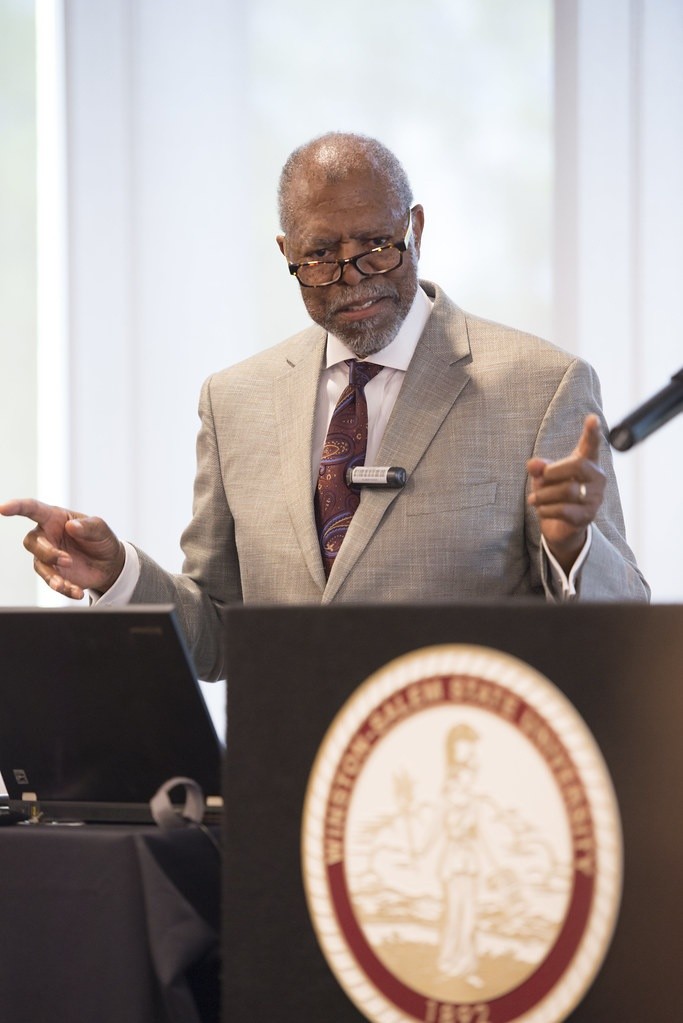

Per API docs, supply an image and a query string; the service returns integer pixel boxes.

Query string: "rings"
[580,484,585,500]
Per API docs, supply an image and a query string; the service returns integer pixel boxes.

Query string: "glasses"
[283,207,413,288]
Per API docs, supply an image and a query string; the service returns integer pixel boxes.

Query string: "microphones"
[607,368,683,451]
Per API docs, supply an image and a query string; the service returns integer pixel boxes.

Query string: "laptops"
[0,606,227,826]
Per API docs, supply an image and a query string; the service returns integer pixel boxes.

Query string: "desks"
[0,818,214,1021]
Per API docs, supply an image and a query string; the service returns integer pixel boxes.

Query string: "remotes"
[344,465,407,489]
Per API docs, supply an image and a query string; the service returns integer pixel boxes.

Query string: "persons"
[0,131,650,683]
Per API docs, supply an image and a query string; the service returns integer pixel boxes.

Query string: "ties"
[313,358,385,584]
